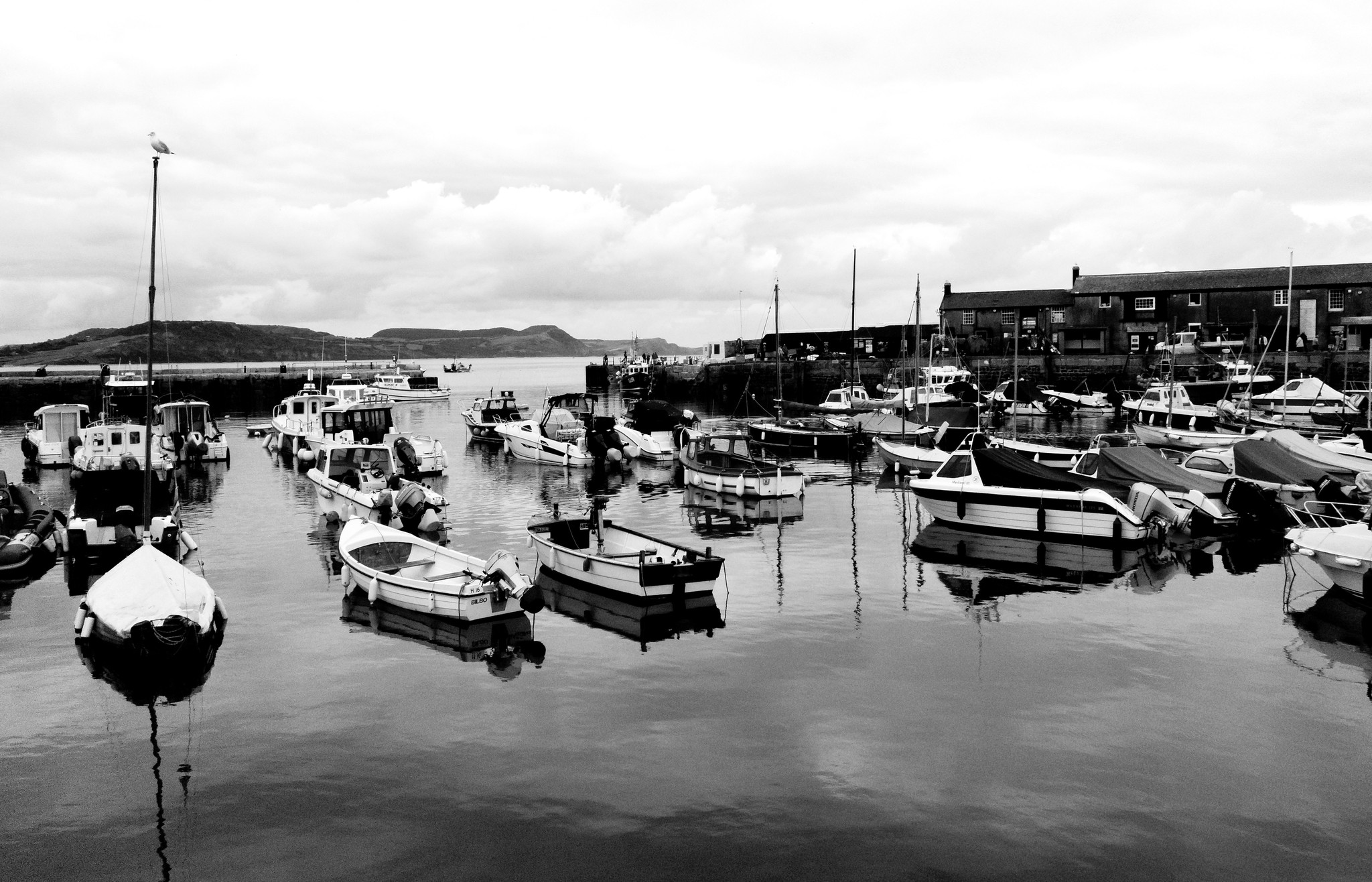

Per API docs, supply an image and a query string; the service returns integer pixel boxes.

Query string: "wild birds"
[148,132,174,158]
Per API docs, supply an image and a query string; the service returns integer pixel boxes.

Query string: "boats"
[337,500,548,622]
[246,328,451,478]
[339,589,547,682]
[525,490,726,596]
[443,357,472,373]
[910,518,1158,608]
[24,403,97,473]
[536,563,726,653]
[0,470,65,586]
[1277,497,1372,601]
[300,430,453,549]
[678,403,806,501]
[463,386,536,443]
[680,483,807,534]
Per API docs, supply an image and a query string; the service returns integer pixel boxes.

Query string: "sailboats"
[65,134,236,667]
[497,249,1372,533]
